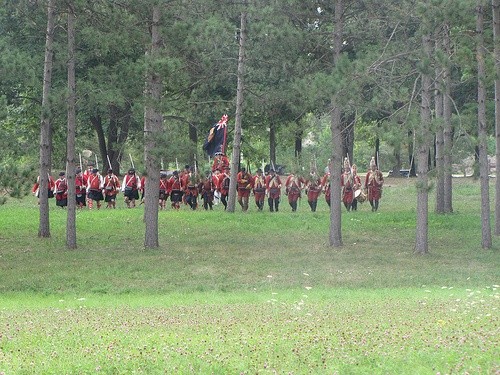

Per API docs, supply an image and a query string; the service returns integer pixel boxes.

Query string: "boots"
[289,201,297,213]
[370,199,379,212]
[268,197,279,212]
[308,201,316,211]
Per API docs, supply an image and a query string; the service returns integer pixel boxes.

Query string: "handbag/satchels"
[172,188,180,195]
[125,186,132,191]
[106,186,113,191]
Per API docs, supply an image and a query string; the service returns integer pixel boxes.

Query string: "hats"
[256,169,263,173]
[204,169,209,174]
[75,169,81,175]
[184,165,189,169]
[173,170,177,175]
[93,169,98,174]
[107,169,112,173]
[240,167,245,172]
[59,172,64,176]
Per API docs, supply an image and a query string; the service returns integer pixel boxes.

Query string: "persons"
[285,169,308,212]
[304,158,384,212]
[141,165,282,212]
[33,167,141,210]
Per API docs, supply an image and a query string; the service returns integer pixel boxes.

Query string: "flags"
[264,164,286,174]
[202,114,227,157]
